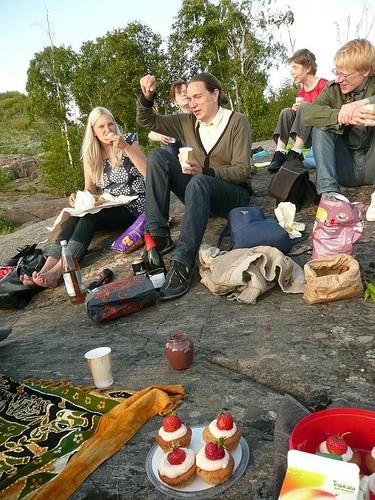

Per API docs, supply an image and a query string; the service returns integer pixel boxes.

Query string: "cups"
[179,145,194,172]
[296,96,304,104]
[84,346,114,387]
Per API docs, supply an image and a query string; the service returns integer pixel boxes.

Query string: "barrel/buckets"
[288,407,374,500]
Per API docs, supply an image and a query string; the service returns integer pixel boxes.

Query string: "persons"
[268,49,328,171]
[20,107,146,288]
[135,72,252,299]
[300,39,375,222]
[148,81,192,161]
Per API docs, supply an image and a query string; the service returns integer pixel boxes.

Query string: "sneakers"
[141,233,175,260]
[283,152,304,167]
[160,260,191,300]
[268,151,284,171]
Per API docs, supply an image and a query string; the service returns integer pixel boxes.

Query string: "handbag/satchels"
[83,267,163,324]
[0,253,47,308]
[217,207,291,254]
[268,160,318,212]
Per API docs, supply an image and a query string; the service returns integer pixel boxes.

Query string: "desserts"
[158,443,197,487]
[156,410,192,452]
[202,410,241,452]
[195,436,234,485]
[318,432,375,500]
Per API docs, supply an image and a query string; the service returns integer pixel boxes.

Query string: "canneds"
[85,268,113,294]
[295,97,305,104]
[130,257,150,280]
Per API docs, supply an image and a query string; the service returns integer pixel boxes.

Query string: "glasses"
[331,67,362,81]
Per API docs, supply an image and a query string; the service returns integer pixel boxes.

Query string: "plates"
[144,424,251,500]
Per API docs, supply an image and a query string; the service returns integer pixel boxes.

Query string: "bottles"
[165,334,194,370]
[141,229,168,292]
[60,240,89,304]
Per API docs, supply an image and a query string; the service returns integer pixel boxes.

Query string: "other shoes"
[365,189,375,222]
